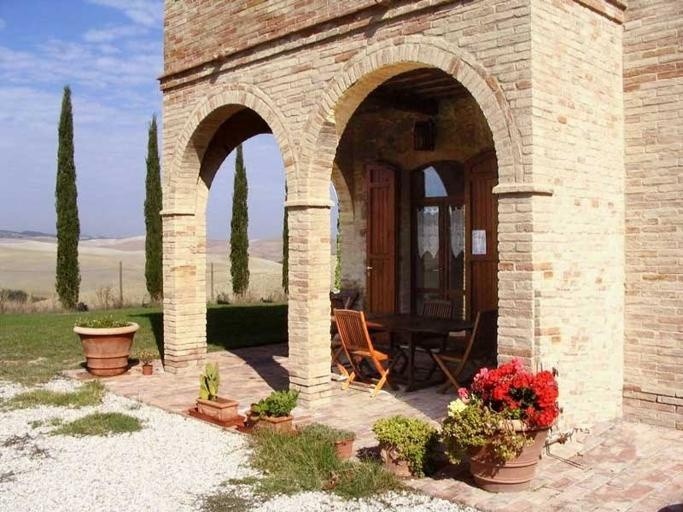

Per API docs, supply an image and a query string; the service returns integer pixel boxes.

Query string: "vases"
[442,431,556,489]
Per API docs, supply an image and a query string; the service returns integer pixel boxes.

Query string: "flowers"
[447,365,563,438]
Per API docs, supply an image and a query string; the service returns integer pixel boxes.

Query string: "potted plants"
[199,368,357,470]
[74,314,153,378]
[375,416,446,477]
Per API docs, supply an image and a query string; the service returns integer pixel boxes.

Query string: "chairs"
[330,284,491,400]
